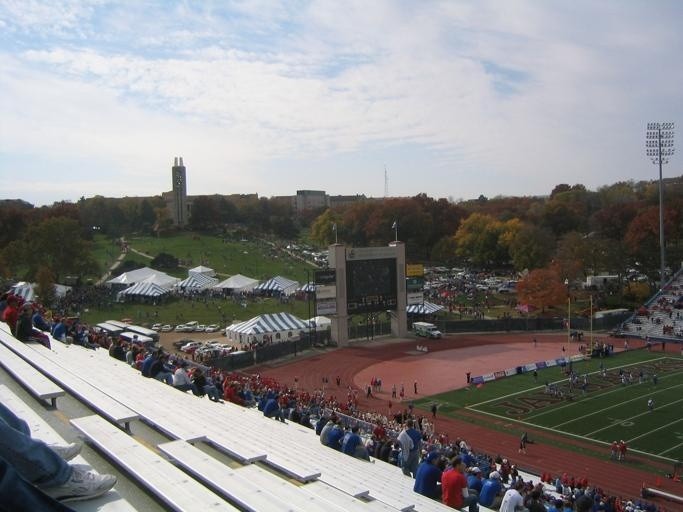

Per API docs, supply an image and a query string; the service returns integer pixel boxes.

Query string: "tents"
[115,265,316,306]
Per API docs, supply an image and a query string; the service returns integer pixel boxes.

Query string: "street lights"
[645,122,675,292]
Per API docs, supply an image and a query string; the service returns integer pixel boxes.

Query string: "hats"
[489,471,500,480]
[471,467,482,474]
[425,451,441,462]
[7,295,19,303]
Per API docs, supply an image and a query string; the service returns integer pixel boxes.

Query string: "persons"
[437,429,666,511]
[425,270,517,323]
[645,396,657,416]
[533,337,536,348]
[98,329,301,426]
[533,369,538,383]
[538,337,659,402]
[0,401,118,505]
[300,372,437,509]
[0,281,106,350]
[613,273,683,337]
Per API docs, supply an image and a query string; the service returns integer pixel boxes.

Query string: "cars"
[619,267,647,286]
[657,267,672,277]
[283,241,330,271]
[151,319,229,333]
[421,264,518,302]
[171,339,250,363]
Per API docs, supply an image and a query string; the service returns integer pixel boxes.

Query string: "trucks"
[585,274,628,289]
[411,319,443,340]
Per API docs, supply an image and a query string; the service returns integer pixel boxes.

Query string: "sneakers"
[35,465,118,507]
[46,442,82,463]
